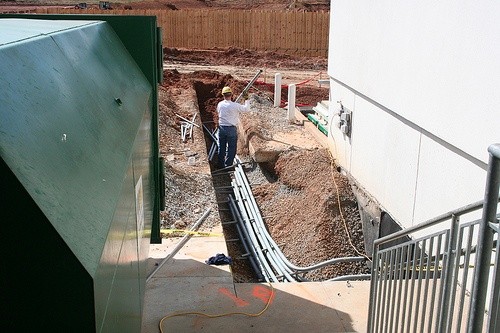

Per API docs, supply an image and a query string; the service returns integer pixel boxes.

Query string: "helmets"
[222,86,232,95]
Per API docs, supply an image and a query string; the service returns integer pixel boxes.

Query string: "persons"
[215,86,251,172]
